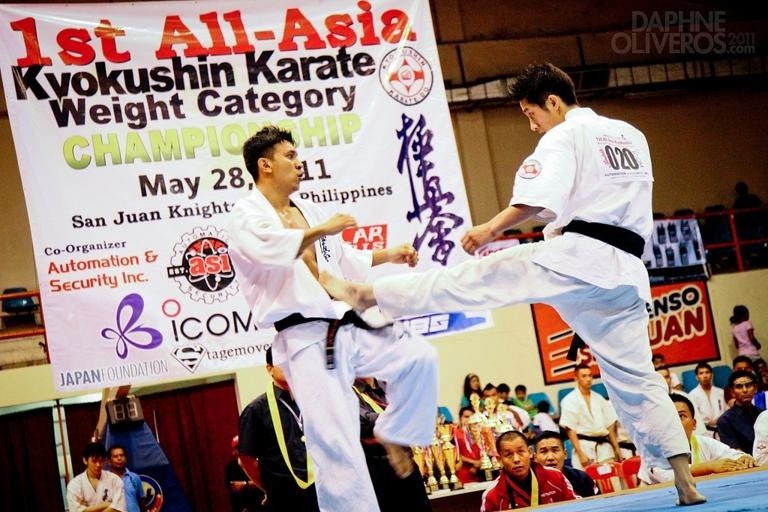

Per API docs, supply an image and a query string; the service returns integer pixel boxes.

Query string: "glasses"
[734,381,754,390]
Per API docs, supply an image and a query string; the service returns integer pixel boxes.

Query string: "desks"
[421,479,494,511]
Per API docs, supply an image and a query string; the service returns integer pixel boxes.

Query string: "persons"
[316,60,706,507]
[237,346,319,512]
[454,305,768,512]
[105,445,147,512]
[224,434,273,512]
[225,126,439,512]
[66,442,127,512]
[732,181,763,241]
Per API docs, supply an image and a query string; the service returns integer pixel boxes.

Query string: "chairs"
[620,453,646,490]
[582,460,626,497]
[432,362,739,469]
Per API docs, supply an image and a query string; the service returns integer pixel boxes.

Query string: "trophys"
[410,413,462,494]
